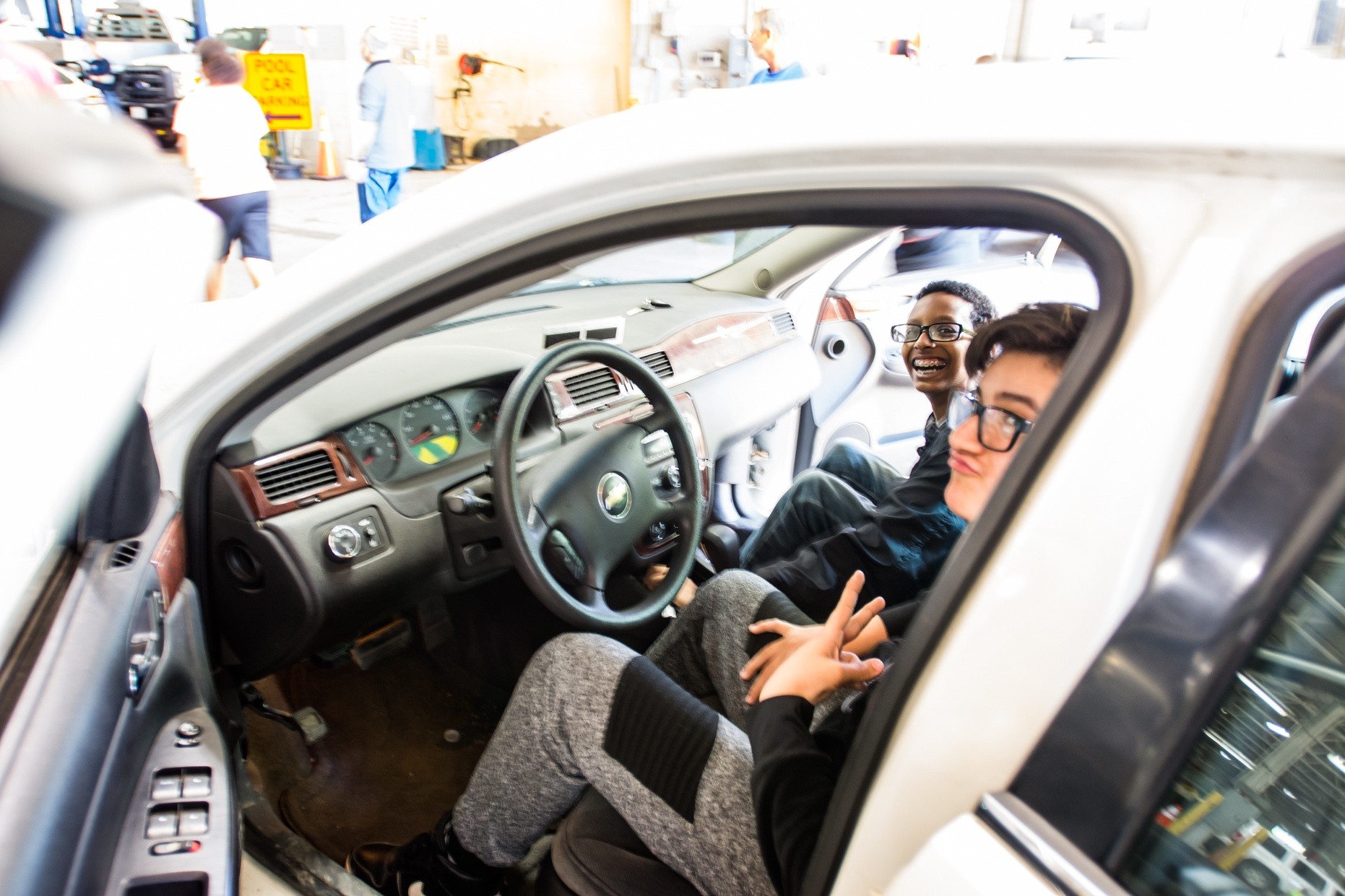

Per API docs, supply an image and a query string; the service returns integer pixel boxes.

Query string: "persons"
[346,305,1105,896]
[170,36,274,300]
[747,7,803,85]
[455,53,513,166]
[350,27,416,222]
[647,282,999,610]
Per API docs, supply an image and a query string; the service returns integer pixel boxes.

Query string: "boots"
[343,810,509,896]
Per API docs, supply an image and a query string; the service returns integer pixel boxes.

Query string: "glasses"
[890,320,976,343]
[945,390,1037,455]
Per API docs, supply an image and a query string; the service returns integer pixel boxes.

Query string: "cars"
[1,25,1345,896]
